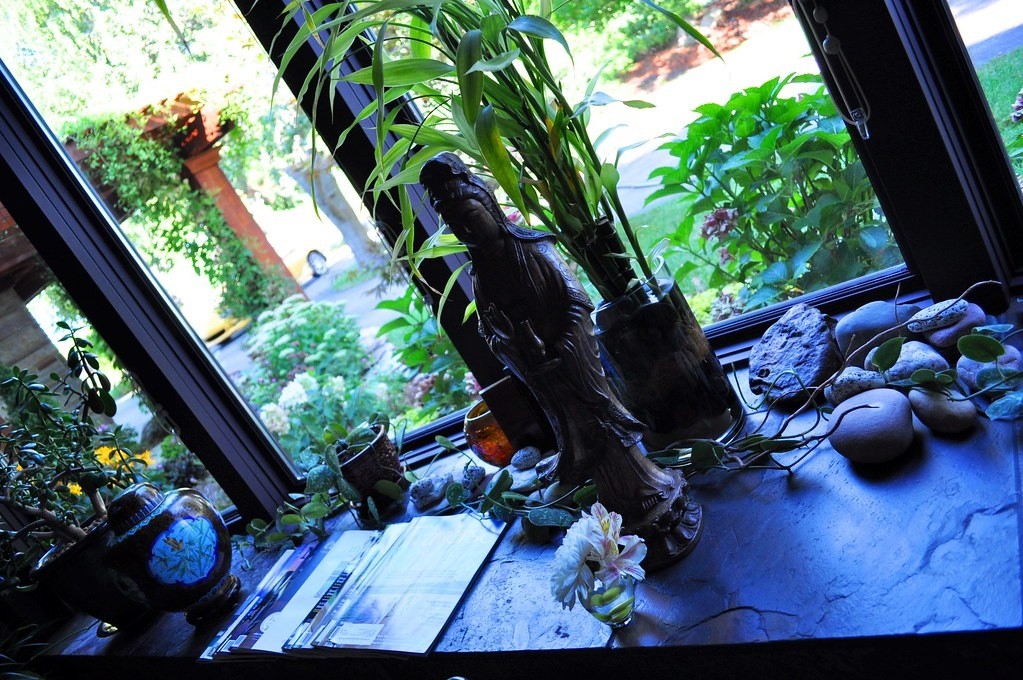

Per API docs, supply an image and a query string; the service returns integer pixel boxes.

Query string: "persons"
[419,151,675,522]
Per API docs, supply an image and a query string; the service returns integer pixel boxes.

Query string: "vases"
[576,568,637,628]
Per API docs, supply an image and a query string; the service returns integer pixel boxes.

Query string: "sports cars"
[195,236,333,349]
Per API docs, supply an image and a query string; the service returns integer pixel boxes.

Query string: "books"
[199,512,511,662]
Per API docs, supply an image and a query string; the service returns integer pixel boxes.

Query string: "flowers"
[549,500,648,611]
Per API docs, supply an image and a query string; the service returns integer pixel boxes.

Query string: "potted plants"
[283,0,746,468]
[298,410,412,504]
[0,323,168,638]
